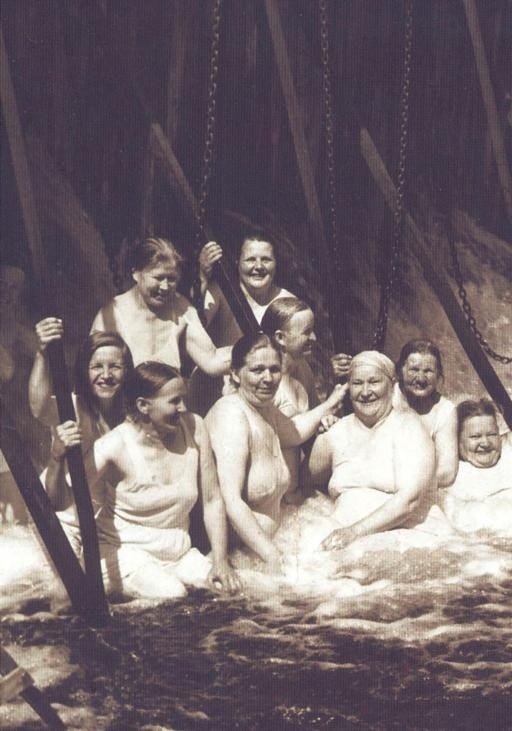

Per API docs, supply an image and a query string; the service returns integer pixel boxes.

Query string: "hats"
[347,350,395,380]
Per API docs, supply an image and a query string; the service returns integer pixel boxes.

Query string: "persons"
[329,337,460,488]
[29,314,132,479]
[258,297,350,430]
[88,238,235,392]
[0,250,29,381]
[201,330,349,571]
[436,398,512,534]
[299,350,437,551]
[189,224,298,346]
[44,360,242,602]
